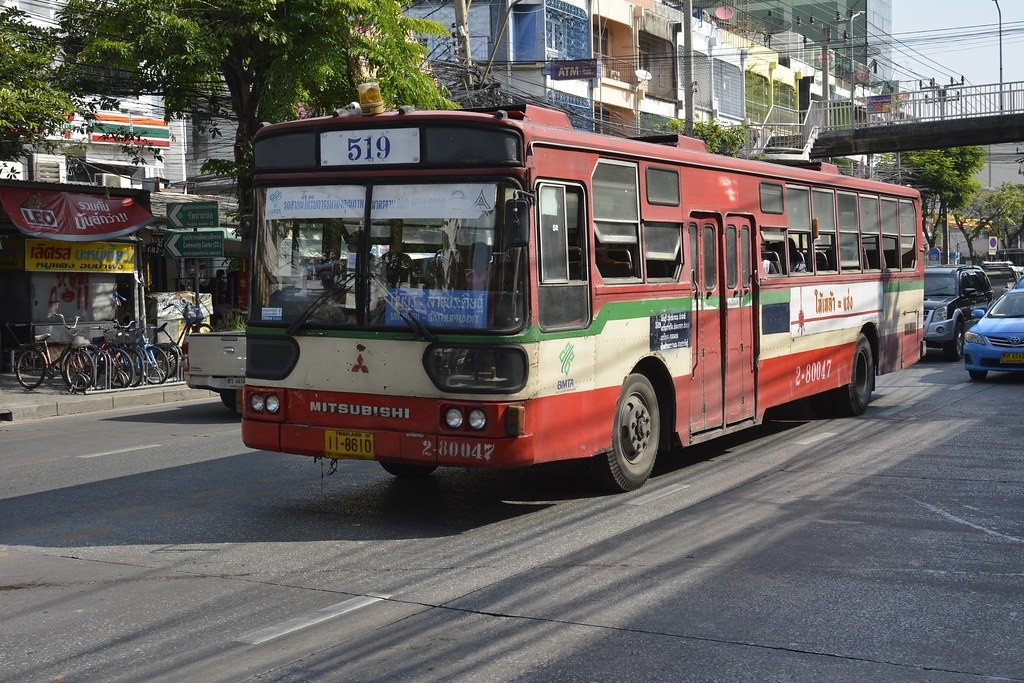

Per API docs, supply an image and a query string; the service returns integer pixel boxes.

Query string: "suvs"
[923,262,996,360]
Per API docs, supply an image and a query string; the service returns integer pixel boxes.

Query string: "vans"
[980,259,1024,301]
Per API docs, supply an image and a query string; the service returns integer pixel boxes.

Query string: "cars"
[962,275,1024,381]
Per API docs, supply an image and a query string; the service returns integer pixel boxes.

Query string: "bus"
[235,84,928,494]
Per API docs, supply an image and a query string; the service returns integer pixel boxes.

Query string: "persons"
[789,238,807,273]
[761,246,777,274]
[207,270,238,326]
[929,278,956,294]
[301,230,389,299]
[448,242,505,329]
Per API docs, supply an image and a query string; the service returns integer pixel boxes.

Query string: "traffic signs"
[165,200,221,230]
[162,227,224,259]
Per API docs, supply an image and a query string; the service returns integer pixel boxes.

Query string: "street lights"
[848,9,868,129]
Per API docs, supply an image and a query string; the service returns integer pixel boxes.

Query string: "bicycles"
[14,293,216,389]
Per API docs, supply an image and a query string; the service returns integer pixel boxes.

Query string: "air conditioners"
[607,69,621,81]
[94,173,132,188]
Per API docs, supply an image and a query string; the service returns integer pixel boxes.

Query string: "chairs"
[761,249,897,275]
[379,250,413,292]
